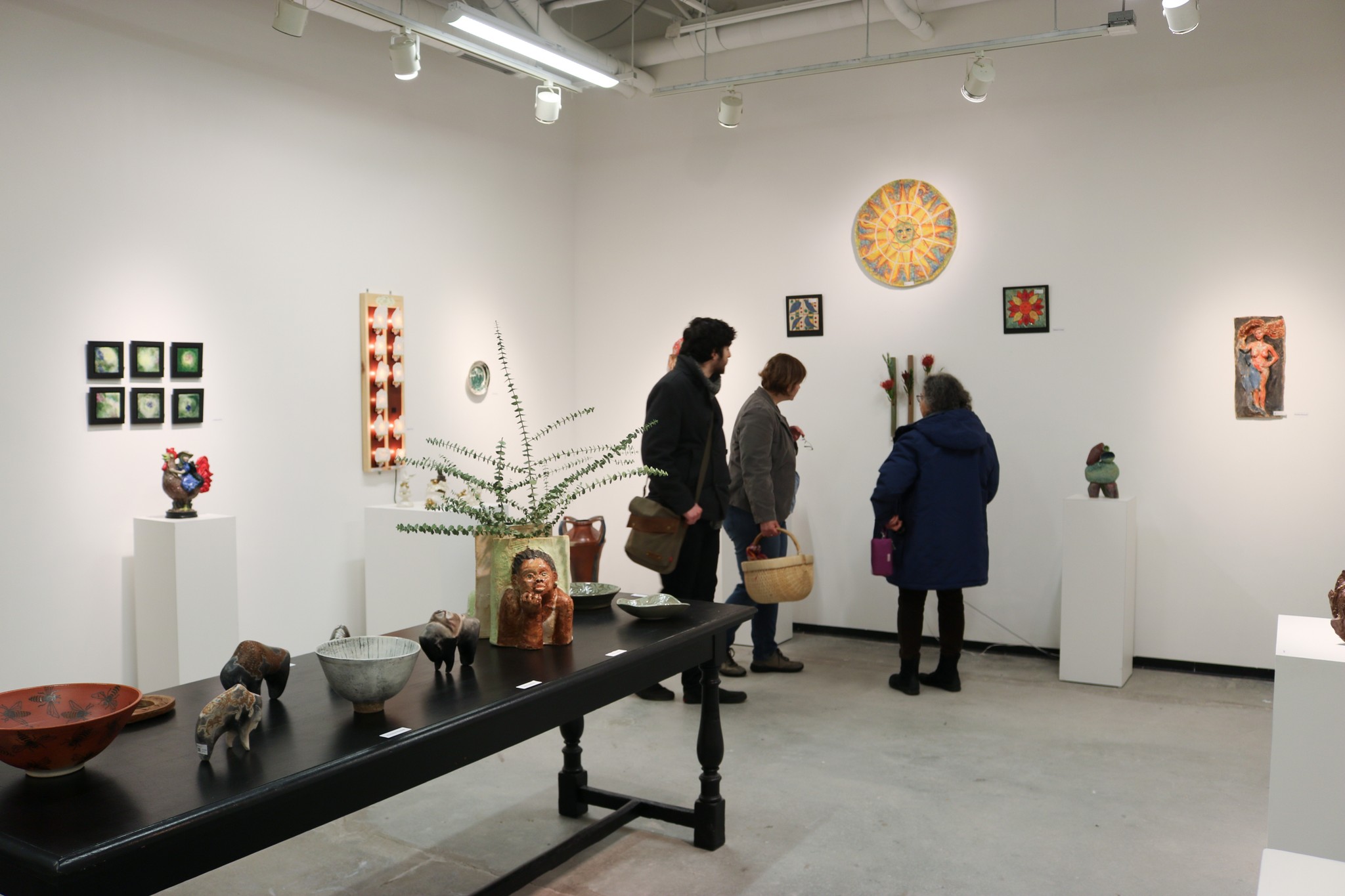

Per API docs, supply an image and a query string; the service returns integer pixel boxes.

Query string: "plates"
[570,582,621,610]
[616,593,691,621]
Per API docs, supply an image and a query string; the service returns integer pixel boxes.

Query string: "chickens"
[161,448,213,511]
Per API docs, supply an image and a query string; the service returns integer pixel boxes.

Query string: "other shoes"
[634,683,675,701]
[889,671,920,696]
[919,671,961,693]
[682,684,746,705]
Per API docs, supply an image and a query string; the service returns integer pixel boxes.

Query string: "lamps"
[442,1,619,88]
[961,50,995,103]
[534,80,562,125]
[718,85,744,129]
[272,0,309,37]
[1161,0,1202,35]
[360,293,405,472]
[388,26,421,81]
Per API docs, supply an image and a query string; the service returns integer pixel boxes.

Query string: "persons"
[497,549,574,649]
[870,373,999,695]
[631,316,748,705]
[720,353,806,678]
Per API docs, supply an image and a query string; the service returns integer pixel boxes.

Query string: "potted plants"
[393,319,669,652]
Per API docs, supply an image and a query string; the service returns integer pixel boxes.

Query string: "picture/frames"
[1003,285,1049,334]
[786,294,823,337]
[88,341,204,425]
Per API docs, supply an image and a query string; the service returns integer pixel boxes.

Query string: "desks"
[1257,615,1345,896]
[1059,495,1137,687]
[133,515,241,693]
[366,502,508,636]
[0,593,758,896]
[713,519,793,647]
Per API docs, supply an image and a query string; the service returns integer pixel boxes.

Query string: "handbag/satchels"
[871,539,892,575]
[624,496,687,575]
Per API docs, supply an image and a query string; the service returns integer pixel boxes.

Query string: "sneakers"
[750,647,804,673]
[718,646,747,677]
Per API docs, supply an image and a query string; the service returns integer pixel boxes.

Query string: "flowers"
[880,353,934,405]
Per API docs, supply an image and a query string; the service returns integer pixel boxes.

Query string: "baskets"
[741,527,815,604]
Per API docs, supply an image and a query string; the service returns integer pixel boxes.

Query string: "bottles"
[558,515,606,583]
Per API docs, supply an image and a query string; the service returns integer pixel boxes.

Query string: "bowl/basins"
[314,635,421,714]
[0,682,143,778]
